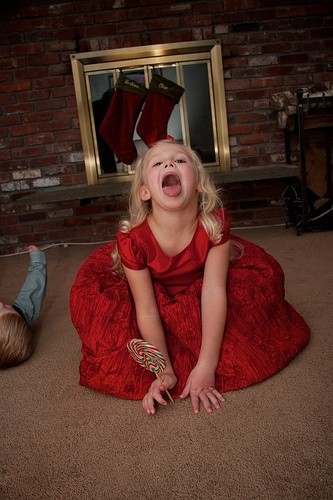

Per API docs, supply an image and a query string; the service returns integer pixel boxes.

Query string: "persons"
[70,140,311,414]
[0,245,47,370]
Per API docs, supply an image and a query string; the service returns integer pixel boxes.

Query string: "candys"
[126,338,174,404]
